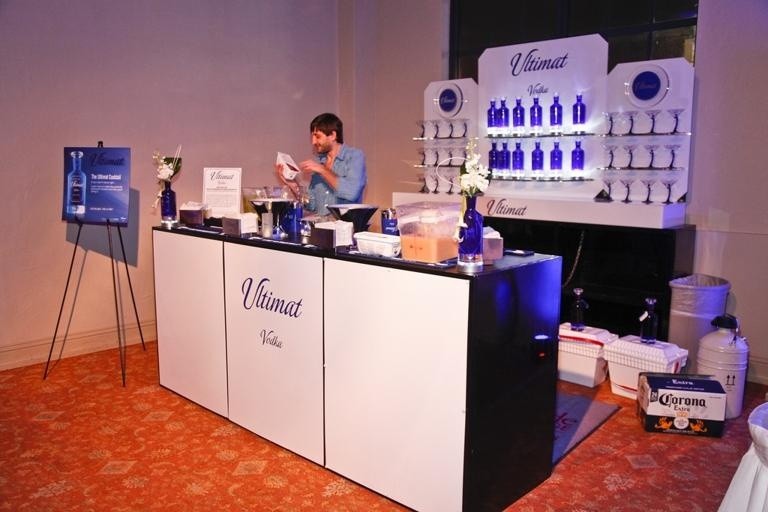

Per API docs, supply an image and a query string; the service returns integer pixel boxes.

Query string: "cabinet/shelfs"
[600,132,685,170]
[484,130,597,183]
[412,135,466,169]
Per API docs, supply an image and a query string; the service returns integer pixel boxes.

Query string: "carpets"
[552,392,621,465]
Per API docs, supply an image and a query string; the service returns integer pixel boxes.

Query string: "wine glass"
[602,176,678,205]
[602,144,682,169]
[415,146,471,168]
[603,108,686,136]
[414,117,471,138]
[414,172,462,195]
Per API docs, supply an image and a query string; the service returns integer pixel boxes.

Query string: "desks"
[151,224,562,512]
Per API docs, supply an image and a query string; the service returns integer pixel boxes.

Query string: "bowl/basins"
[248,198,295,237]
[327,203,381,230]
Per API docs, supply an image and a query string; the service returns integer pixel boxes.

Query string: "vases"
[161,181,177,220]
[457,197,483,273]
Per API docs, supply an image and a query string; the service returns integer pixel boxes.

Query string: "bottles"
[66,151,87,215]
[486,92,586,137]
[569,288,587,330]
[638,298,660,344]
[487,138,586,182]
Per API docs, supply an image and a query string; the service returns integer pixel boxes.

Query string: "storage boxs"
[557,321,618,389]
[399,235,459,263]
[603,335,688,401]
[636,370,728,437]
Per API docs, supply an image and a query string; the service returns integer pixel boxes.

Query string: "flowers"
[436,136,491,242]
[152,144,182,208]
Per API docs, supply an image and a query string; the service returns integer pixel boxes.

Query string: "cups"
[261,213,273,238]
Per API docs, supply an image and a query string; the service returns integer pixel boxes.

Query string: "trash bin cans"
[667,273,732,379]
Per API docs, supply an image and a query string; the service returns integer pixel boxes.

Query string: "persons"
[275,112,368,218]
